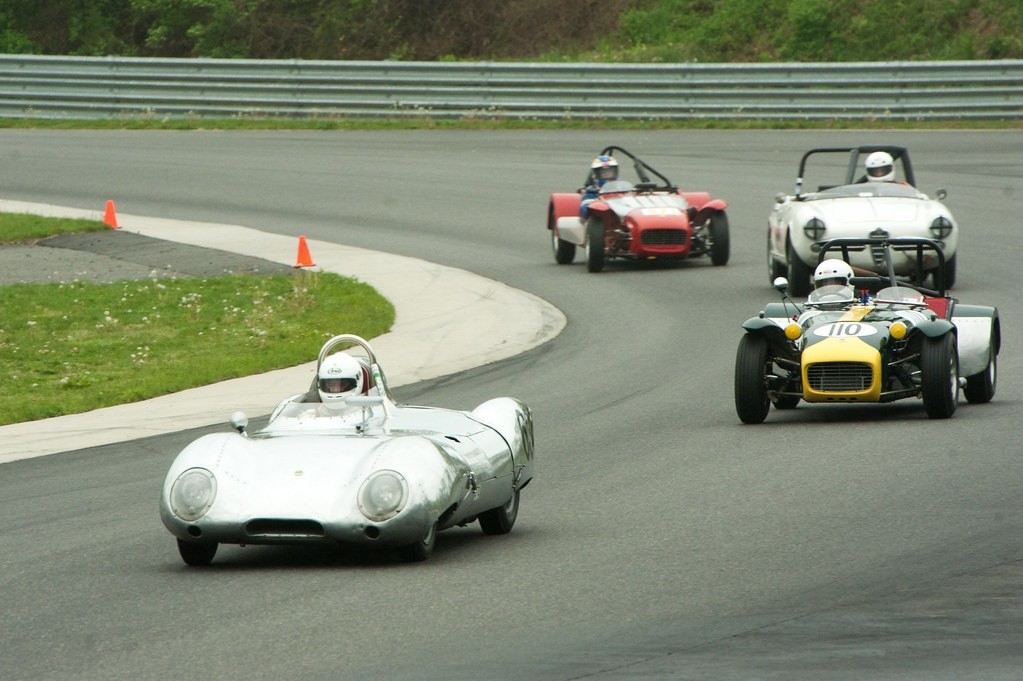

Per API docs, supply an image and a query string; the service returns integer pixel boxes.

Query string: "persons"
[865,151,908,184]
[813,259,856,299]
[298,352,365,419]
[579,155,622,216]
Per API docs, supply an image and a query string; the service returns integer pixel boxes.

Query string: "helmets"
[814,259,855,292]
[591,155,619,187]
[865,151,896,182]
[317,353,364,403]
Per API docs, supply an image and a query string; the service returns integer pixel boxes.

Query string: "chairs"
[317,334,375,393]
[877,286,924,307]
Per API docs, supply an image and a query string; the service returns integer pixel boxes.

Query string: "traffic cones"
[292,236,315,268]
[101,199,122,229]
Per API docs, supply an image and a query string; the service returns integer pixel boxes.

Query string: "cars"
[767,145,958,297]
[160,333,536,566]
[735,237,1002,424]
[547,146,731,273]
